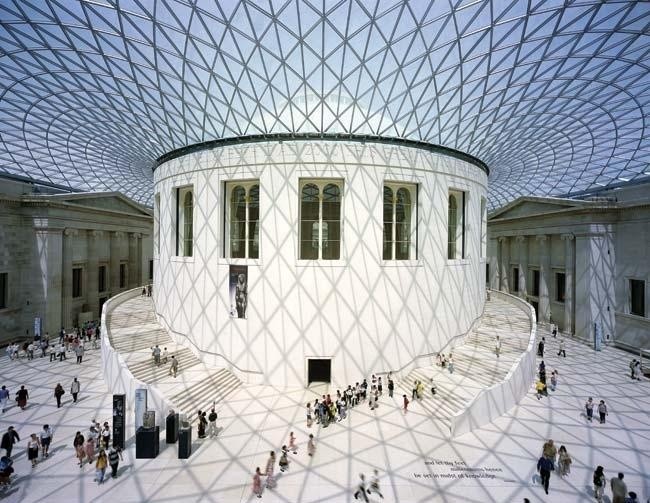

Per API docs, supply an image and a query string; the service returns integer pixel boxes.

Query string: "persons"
[236,274,247,319]
